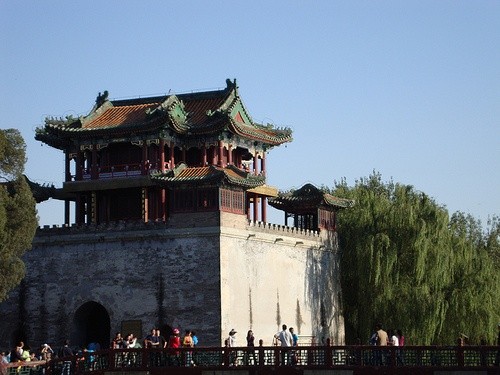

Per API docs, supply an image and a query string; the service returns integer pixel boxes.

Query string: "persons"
[274,324,299,366]
[1,340,100,375]
[226,329,238,366]
[246,330,257,365]
[183,330,198,367]
[112,332,137,367]
[370,323,405,367]
[167,328,181,366]
[142,328,167,367]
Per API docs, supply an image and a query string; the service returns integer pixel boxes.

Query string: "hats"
[173,329,180,334]
[230,329,237,333]
[44,344,48,347]
